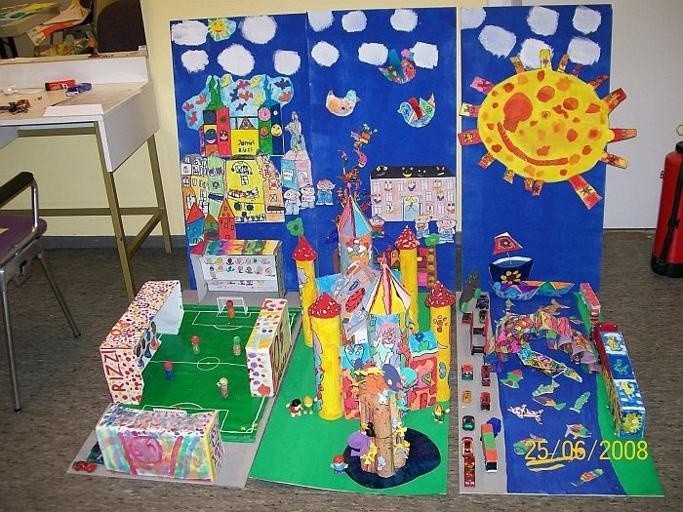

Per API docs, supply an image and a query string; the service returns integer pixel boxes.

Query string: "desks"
[0,0,61,60]
[0,83,173,308]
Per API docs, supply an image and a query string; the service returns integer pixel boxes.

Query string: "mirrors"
[0,0,148,62]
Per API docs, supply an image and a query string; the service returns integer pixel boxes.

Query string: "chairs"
[47,0,100,56]
[0,170,81,414]
[93,1,143,52]
